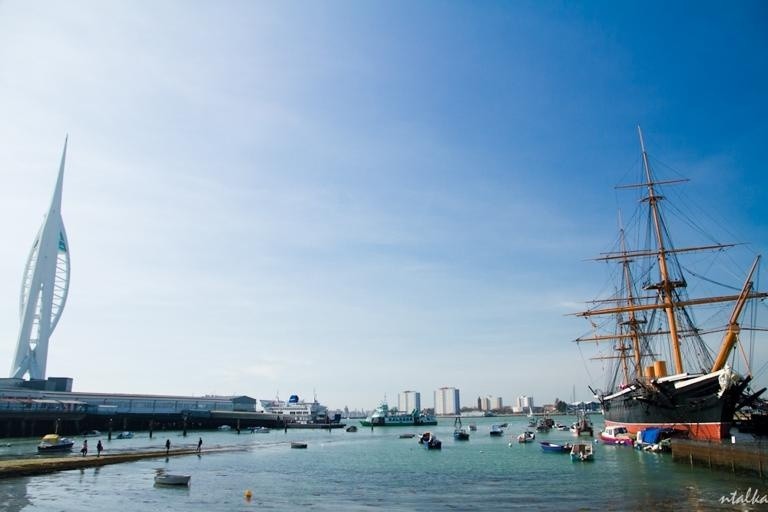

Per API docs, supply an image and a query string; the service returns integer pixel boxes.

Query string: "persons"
[79,439,89,456]
[163,438,171,451]
[196,435,204,451]
[96,440,102,457]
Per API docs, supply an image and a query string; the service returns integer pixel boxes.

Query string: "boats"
[116,430,135,439]
[82,429,102,438]
[288,441,307,449]
[215,423,269,434]
[344,403,678,466]
[150,470,191,487]
[36,432,75,454]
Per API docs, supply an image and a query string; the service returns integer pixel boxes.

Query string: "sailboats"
[560,123,767,448]
